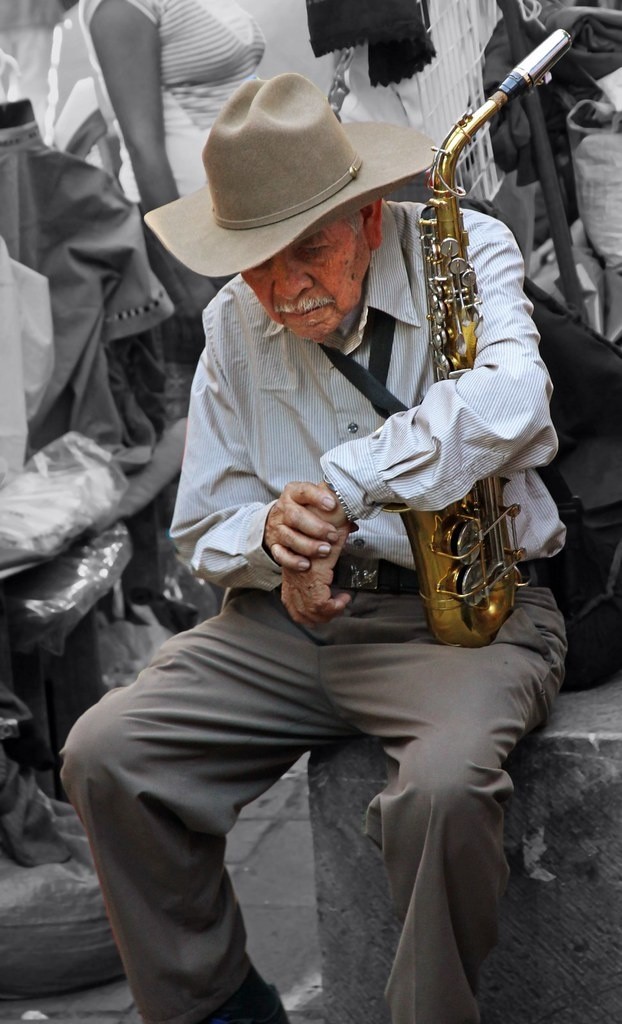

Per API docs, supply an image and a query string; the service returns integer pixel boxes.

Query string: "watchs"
[323,472,356,523]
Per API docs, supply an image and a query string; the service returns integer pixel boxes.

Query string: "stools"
[0,522,132,804]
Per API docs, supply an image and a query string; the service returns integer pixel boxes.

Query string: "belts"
[334,556,416,592]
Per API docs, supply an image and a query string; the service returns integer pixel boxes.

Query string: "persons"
[58,75,568,1024]
[0,0,622,882]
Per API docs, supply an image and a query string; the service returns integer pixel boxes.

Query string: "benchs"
[307,672,621,1024]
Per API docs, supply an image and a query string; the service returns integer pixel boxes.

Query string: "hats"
[144,72,439,277]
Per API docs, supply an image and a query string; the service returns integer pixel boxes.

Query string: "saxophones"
[374,23,575,650]
